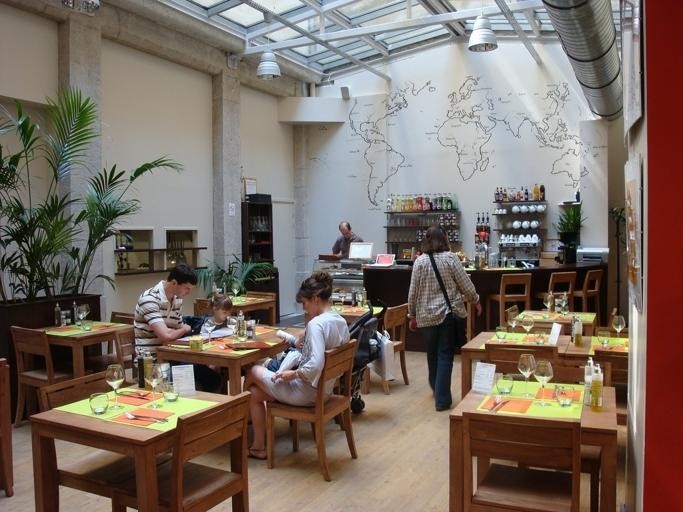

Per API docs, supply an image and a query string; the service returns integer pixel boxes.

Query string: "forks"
[488,396,501,412]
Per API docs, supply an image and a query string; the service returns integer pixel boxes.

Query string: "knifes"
[494,401,510,415]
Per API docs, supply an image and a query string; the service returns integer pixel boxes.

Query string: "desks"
[364,261,608,352]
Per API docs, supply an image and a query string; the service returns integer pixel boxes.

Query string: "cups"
[519,354,536,399]
[356,292,363,312]
[77,306,87,330]
[555,300,555,315]
[496,327,507,338]
[508,313,521,339]
[545,294,554,314]
[532,362,555,407]
[612,316,624,342]
[533,331,545,343]
[161,383,179,403]
[107,365,125,409]
[336,302,343,312]
[338,290,346,311]
[89,394,109,414]
[520,316,534,341]
[554,387,575,406]
[597,333,609,342]
[206,318,216,345]
[227,317,240,342]
[497,376,512,394]
[363,301,372,310]
[145,365,162,408]
[80,321,93,331]
[542,309,551,318]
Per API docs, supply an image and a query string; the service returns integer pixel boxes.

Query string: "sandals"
[246,447,267,460]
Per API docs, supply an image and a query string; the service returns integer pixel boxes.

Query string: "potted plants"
[553,207,589,264]
[0,86,184,423]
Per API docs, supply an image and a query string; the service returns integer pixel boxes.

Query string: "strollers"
[346,297,387,413]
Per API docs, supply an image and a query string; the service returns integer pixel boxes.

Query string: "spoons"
[124,411,166,422]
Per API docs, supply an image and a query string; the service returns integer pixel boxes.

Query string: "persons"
[262,330,306,382]
[134,265,221,393]
[243,272,350,459]
[200,294,238,393]
[332,221,362,260]
[407,225,482,411]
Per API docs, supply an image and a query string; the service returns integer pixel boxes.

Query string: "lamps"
[256,50,282,80]
[468,16,498,52]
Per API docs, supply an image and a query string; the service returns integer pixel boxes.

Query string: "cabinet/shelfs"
[114,247,209,276]
[383,210,461,259]
[313,258,369,305]
[492,201,548,267]
[241,201,279,324]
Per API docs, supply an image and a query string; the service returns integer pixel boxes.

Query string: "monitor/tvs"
[348,242,373,260]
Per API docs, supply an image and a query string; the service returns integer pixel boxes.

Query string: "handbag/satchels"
[429,255,468,353]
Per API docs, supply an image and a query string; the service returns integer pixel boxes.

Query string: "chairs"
[112,391,249,512]
[381,302,409,393]
[535,272,577,312]
[115,328,135,369]
[38,371,113,413]
[573,270,601,326]
[518,357,612,512]
[505,305,518,328]
[485,342,558,381]
[194,298,213,317]
[0,357,14,497]
[88,311,135,371]
[10,325,71,428]
[267,339,358,482]
[245,291,276,328]
[461,412,580,512]
[486,274,531,332]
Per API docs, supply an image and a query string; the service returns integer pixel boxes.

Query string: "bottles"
[519,185,524,201]
[503,188,507,201]
[584,381,590,406]
[237,310,245,337]
[473,232,490,269]
[276,330,296,345]
[71,302,78,324]
[66,310,72,326]
[590,364,603,412]
[495,187,499,201]
[517,191,520,201]
[387,192,457,210]
[528,185,533,201]
[571,316,576,343]
[525,189,528,200]
[575,323,582,347]
[540,185,545,202]
[584,357,594,393]
[54,303,62,326]
[533,184,539,200]
[476,212,490,234]
[61,311,66,325]
[247,324,253,339]
[143,351,153,391]
[137,351,144,388]
[499,186,503,201]
